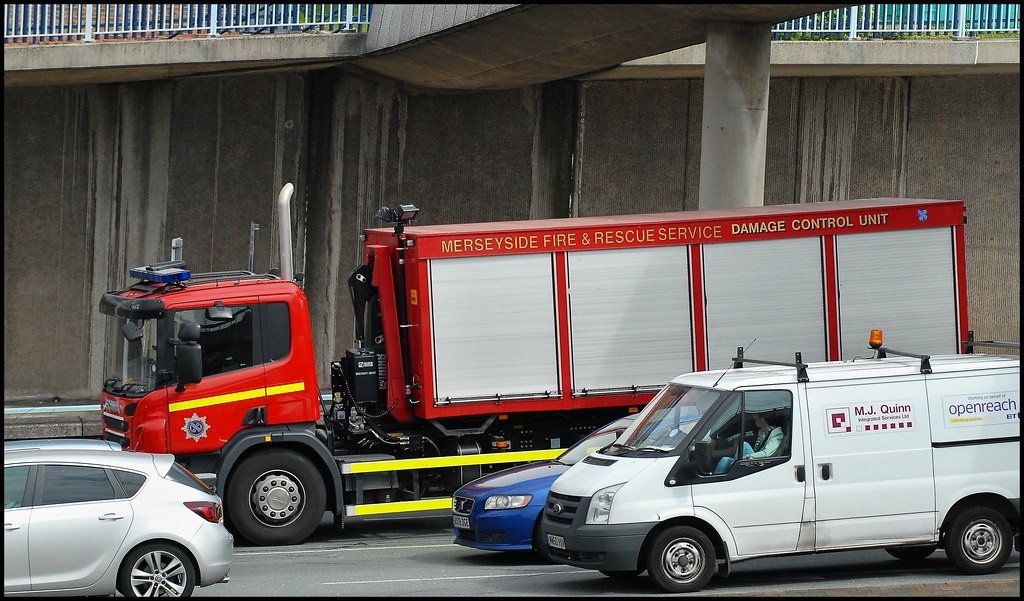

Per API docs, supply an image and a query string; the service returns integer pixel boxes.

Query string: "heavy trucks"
[98,199,973,545]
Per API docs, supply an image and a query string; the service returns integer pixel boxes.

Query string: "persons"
[709,405,784,474]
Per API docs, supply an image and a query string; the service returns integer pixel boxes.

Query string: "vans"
[540,332,1020,593]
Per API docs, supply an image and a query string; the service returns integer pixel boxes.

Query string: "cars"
[4,438,234,598]
[454,407,699,562]
